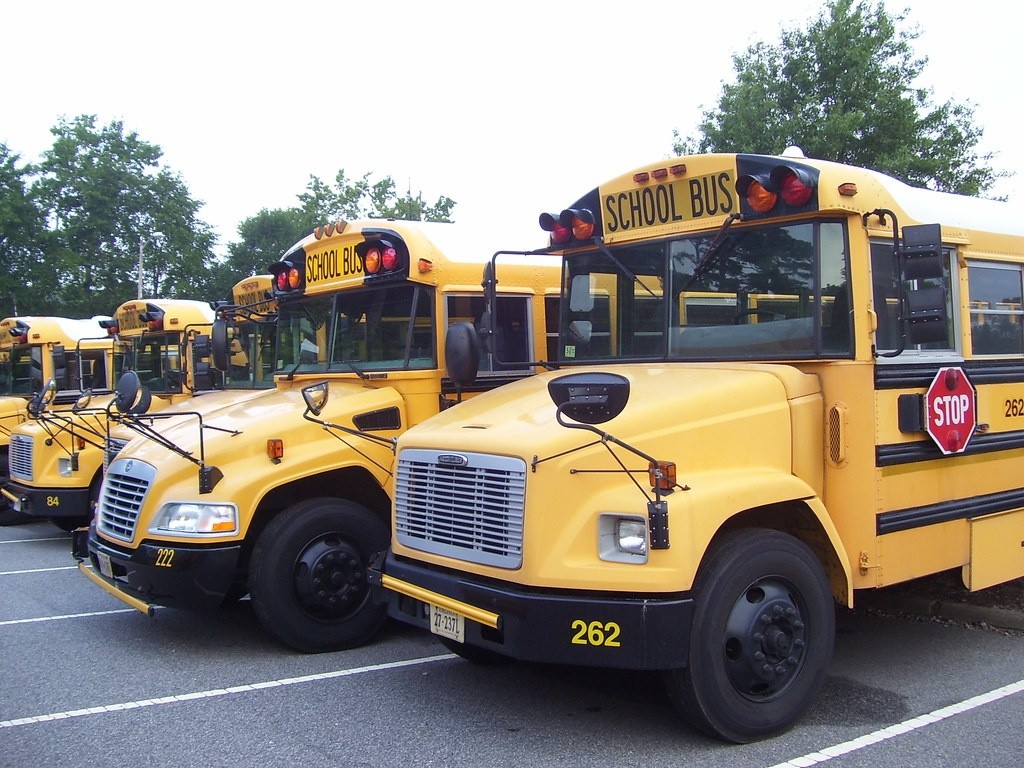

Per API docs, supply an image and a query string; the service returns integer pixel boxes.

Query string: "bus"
[70,271,477,473]
[0,314,119,528]
[70,215,836,654]
[379,151,1024,746]
[0,296,242,533]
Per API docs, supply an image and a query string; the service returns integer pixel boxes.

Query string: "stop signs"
[923,366,976,456]
